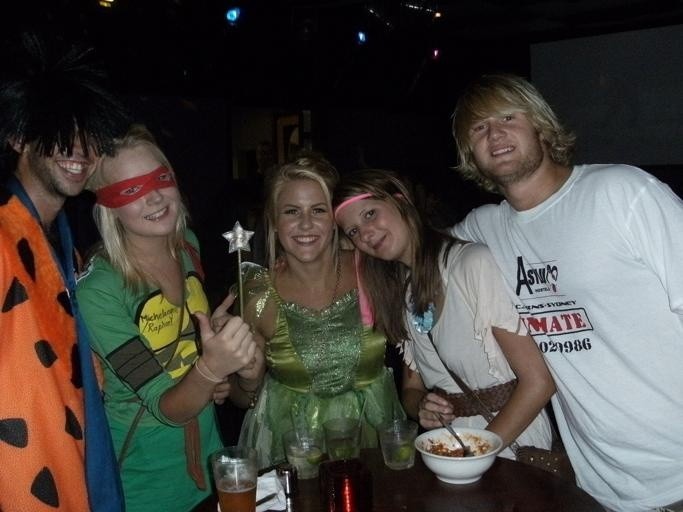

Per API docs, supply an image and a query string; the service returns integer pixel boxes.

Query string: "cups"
[282,428,325,480]
[377,419,418,470]
[323,418,362,461]
[209,447,258,512]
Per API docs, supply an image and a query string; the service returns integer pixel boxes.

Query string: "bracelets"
[190,355,229,384]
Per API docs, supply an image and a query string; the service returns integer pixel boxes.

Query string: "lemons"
[307,447,322,465]
[391,443,413,462]
[333,444,357,460]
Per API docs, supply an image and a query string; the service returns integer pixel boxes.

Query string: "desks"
[190,448,605,512]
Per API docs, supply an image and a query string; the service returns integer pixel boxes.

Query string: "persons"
[210,157,407,472]
[0,91,115,511]
[74,120,257,511]
[434,76,683,512]
[331,170,556,462]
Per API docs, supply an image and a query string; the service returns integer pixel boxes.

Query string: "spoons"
[433,410,474,457]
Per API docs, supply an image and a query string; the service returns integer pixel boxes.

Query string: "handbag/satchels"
[427,329,573,481]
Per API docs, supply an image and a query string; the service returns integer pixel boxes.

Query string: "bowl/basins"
[414,428,503,485]
